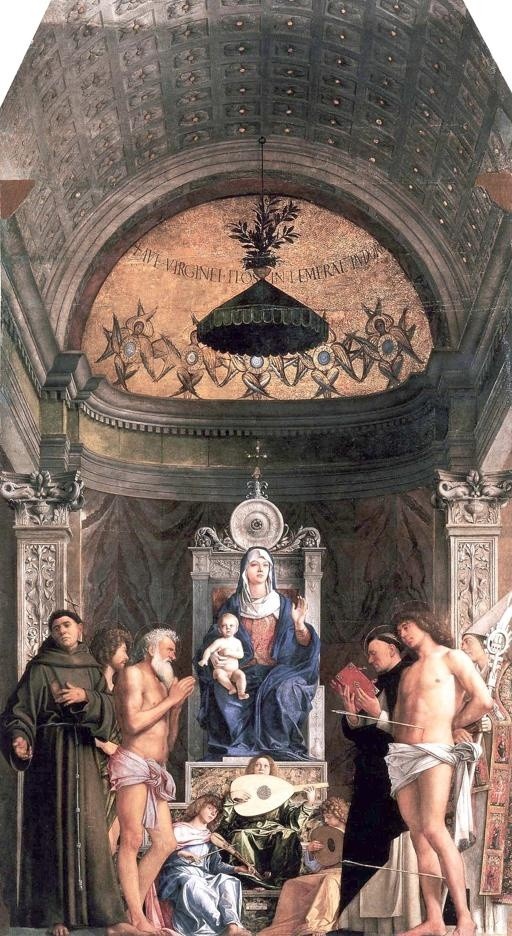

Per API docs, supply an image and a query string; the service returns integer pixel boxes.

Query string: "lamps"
[197,136,329,357]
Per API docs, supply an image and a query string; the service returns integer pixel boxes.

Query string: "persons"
[0,547,512,935]
